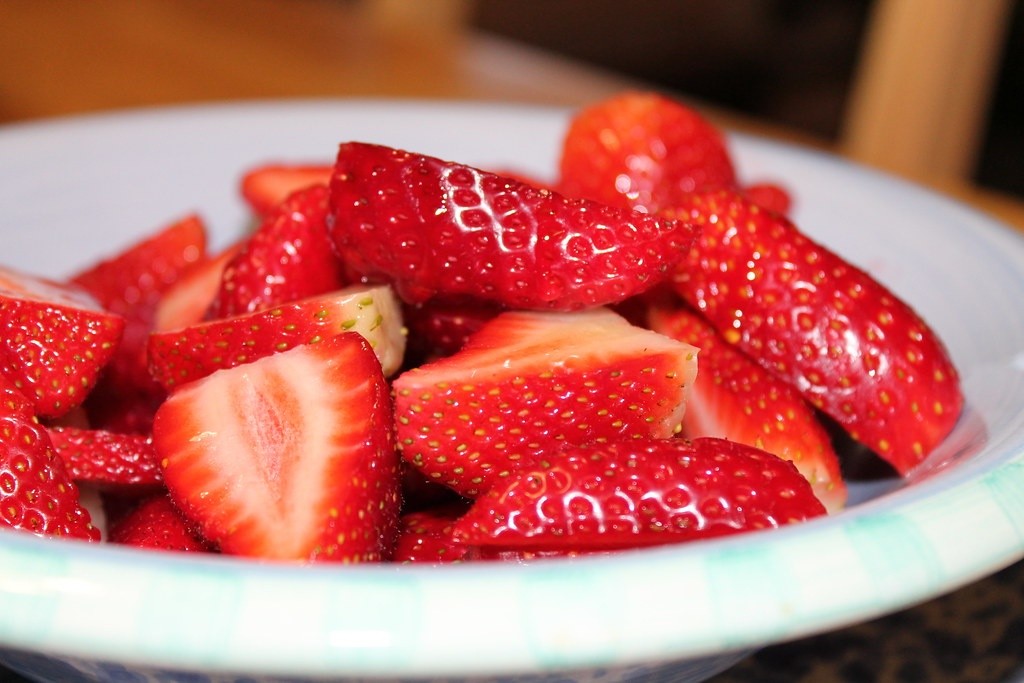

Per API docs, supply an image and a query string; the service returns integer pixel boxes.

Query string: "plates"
[0,97,1024,683]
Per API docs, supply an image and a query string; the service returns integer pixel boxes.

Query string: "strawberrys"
[0,86,963,563]
